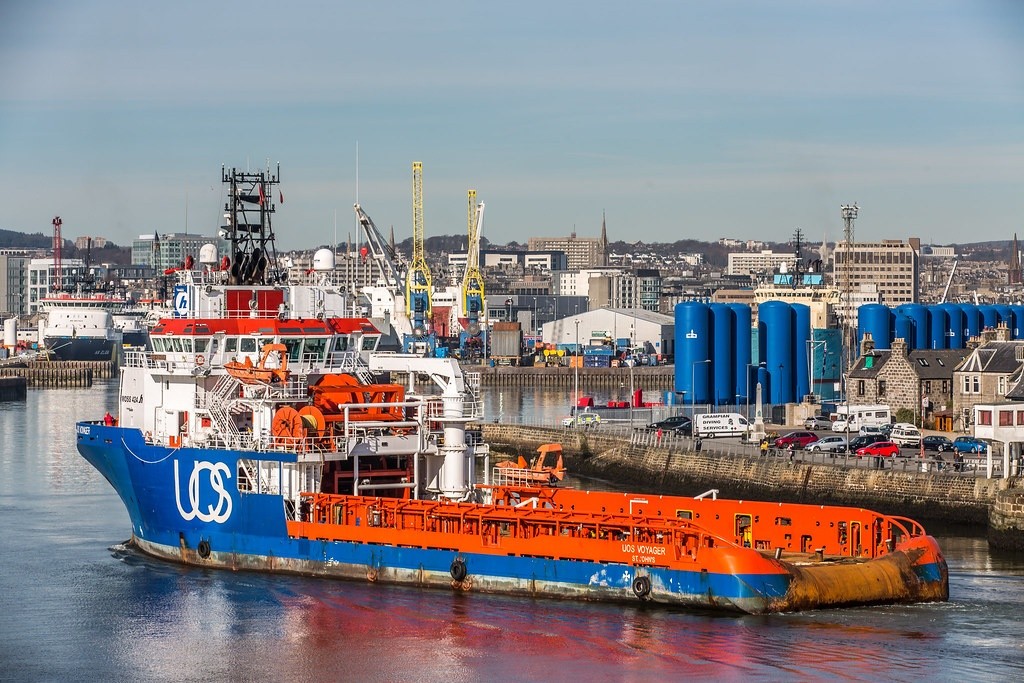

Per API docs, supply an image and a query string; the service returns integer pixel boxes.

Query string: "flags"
[258,184,264,206]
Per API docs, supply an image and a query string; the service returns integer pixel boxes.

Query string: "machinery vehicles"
[459,188,491,361]
[401,159,449,358]
[51,217,63,292]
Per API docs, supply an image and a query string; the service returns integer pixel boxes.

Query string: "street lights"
[778,364,785,426]
[830,363,838,415]
[553,298,556,321]
[683,360,711,440]
[845,369,869,460]
[735,362,767,448]
[533,297,536,338]
[508,297,512,323]
[574,305,578,314]
[572,317,581,428]
[585,297,589,312]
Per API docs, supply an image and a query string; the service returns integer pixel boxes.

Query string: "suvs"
[562,413,601,428]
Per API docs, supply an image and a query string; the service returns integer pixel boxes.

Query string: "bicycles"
[766,449,784,457]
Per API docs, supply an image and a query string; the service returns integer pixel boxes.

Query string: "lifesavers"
[450,561,467,581]
[222,256,230,269]
[186,255,193,268]
[196,354,205,365]
[633,577,650,597]
[198,541,210,558]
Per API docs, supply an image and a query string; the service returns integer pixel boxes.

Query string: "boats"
[495,444,567,481]
[70,167,953,617]
[225,344,291,384]
[39,236,148,360]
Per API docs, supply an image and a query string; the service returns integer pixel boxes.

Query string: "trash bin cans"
[696,438,702,451]
[874,457,884,468]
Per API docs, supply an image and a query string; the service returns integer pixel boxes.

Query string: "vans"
[832,409,891,434]
[693,414,755,439]
[889,424,919,446]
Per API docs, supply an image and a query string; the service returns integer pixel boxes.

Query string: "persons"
[936,452,944,469]
[761,440,768,456]
[789,444,795,464]
[954,450,964,471]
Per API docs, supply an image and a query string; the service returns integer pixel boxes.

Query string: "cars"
[952,436,987,453]
[772,425,901,458]
[805,416,832,430]
[920,436,953,453]
[645,417,692,436]
